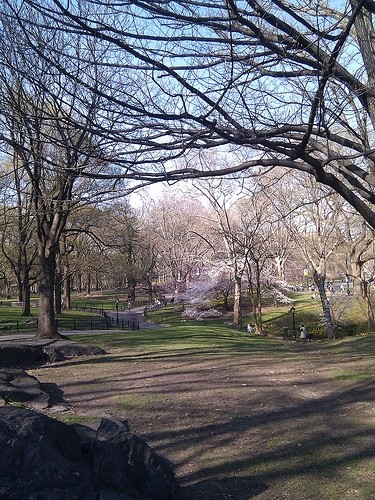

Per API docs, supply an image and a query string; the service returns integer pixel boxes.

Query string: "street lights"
[291,307,296,340]
